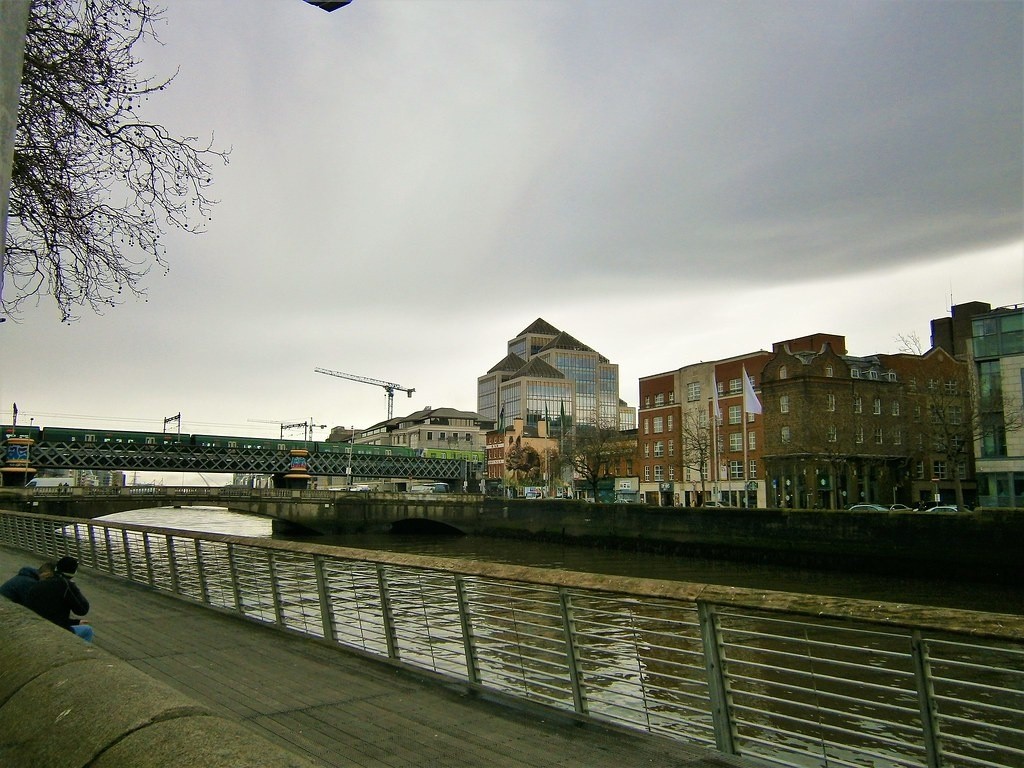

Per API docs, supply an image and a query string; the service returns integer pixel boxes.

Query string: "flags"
[742,363,762,415]
[711,370,720,419]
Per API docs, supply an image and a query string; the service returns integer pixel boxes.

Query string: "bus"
[411,482,449,493]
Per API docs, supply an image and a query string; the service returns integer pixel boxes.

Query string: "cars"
[847,503,972,512]
[700,500,737,507]
[613,498,635,503]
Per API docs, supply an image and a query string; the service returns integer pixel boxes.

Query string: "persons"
[0,556,95,645]
[58,483,69,496]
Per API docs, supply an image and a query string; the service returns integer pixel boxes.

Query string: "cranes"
[314,366,416,420]
[247,417,327,442]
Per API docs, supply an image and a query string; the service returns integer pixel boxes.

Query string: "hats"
[55,557,77,577]
